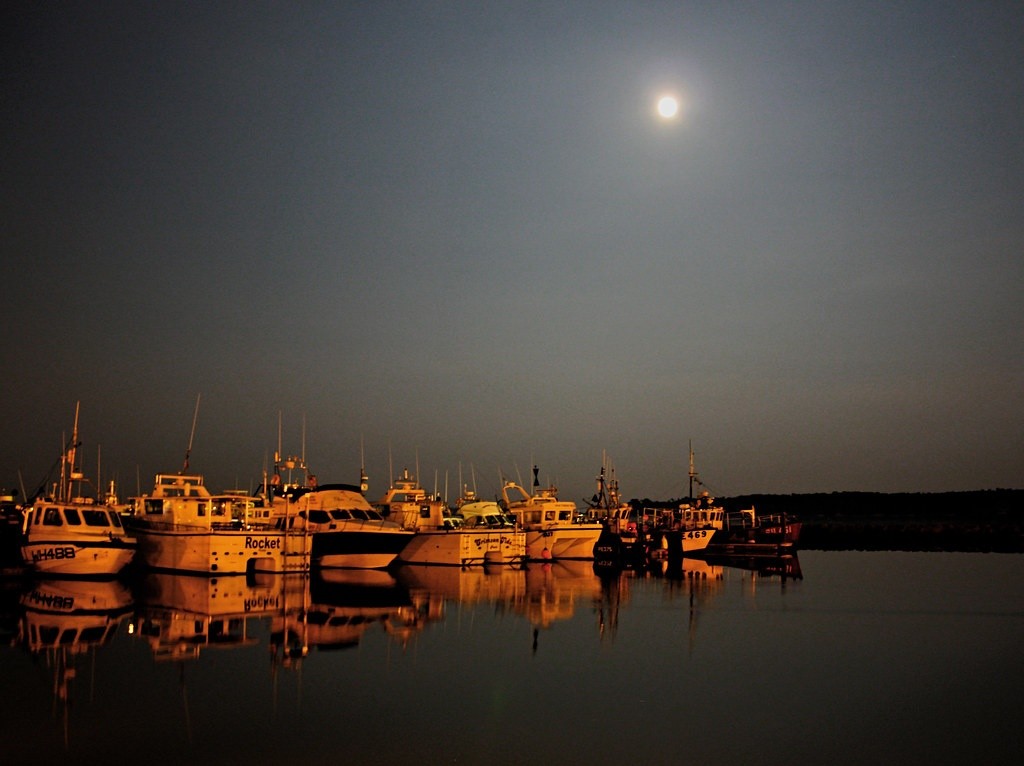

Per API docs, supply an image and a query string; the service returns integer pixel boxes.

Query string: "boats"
[10,390,806,579]
[9,579,427,752]
